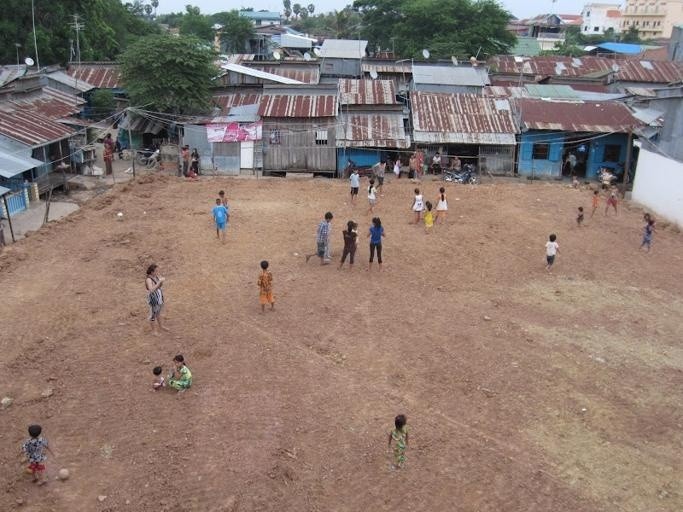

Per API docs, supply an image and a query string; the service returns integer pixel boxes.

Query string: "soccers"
[59,469,69,479]
[116,213,122,218]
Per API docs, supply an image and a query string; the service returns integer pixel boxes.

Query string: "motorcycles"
[136,143,161,164]
[443,163,479,185]
[598,160,631,182]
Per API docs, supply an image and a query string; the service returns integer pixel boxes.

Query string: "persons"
[339,157,387,212]
[388,414,410,468]
[638,219,659,251]
[151,355,192,392]
[15,425,56,485]
[219,191,227,218]
[103,132,115,174]
[590,192,601,217]
[256,261,275,315]
[321,212,333,262]
[352,224,358,240]
[337,220,357,271]
[211,198,230,244]
[409,186,447,233]
[392,149,463,176]
[575,207,584,227]
[146,265,169,337]
[179,145,201,176]
[366,217,386,272]
[543,234,562,273]
[603,185,618,215]
